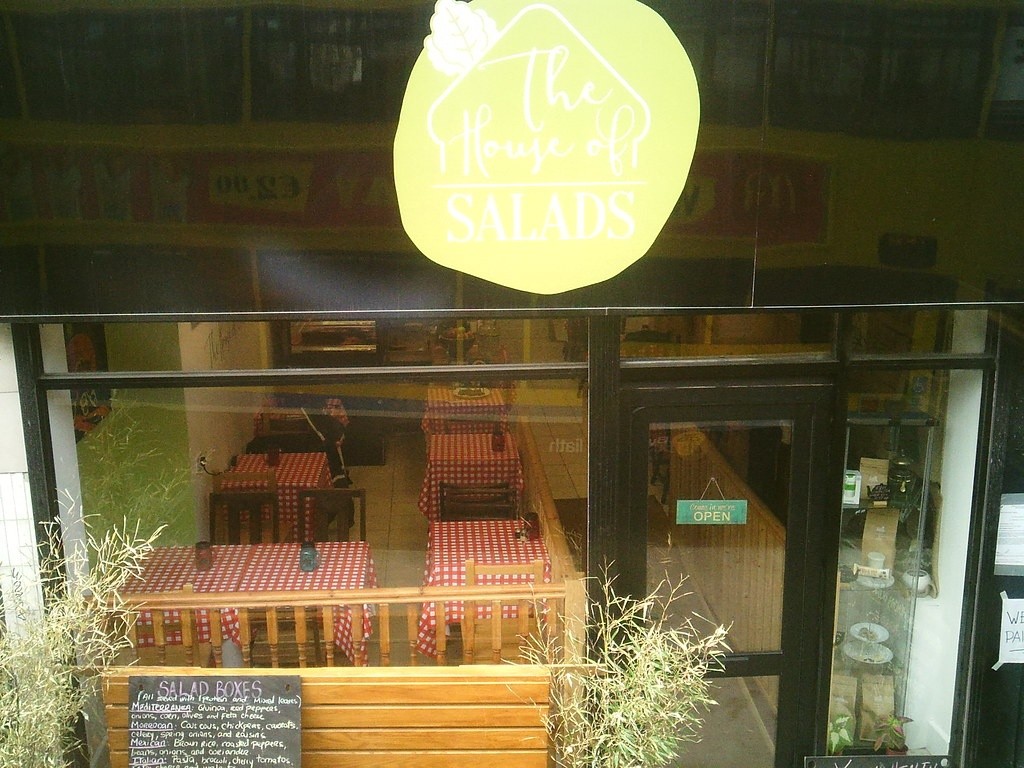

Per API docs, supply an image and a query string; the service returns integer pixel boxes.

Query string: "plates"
[850,623,889,643]
[453,387,490,397]
[844,640,894,664]
[856,575,894,589]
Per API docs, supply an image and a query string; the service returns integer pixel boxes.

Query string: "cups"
[525,512,539,539]
[263,445,279,465]
[867,551,884,570]
[903,569,937,597]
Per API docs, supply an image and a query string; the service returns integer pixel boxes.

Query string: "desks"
[115,540,372,668]
[219,452,326,541]
[433,521,551,644]
[427,388,506,433]
[258,398,341,449]
[429,433,520,519]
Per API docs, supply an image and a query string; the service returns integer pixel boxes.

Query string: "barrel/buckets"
[442,337,474,358]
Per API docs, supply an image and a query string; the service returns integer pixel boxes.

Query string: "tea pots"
[866,483,892,501]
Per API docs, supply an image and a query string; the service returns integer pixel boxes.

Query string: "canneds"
[195,542,213,570]
[493,432,504,452]
[301,542,317,571]
[525,512,539,538]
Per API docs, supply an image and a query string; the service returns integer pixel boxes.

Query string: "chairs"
[460,560,545,664]
[212,468,294,544]
[208,492,280,544]
[439,481,510,519]
[263,412,315,436]
[248,605,326,667]
[298,488,367,541]
[445,488,516,520]
[443,405,500,432]
[108,582,214,668]
[650,422,671,505]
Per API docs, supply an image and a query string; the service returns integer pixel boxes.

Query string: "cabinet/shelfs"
[845,410,944,749]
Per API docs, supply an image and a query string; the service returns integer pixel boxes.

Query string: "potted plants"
[826,714,853,755]
[873,710,914,755]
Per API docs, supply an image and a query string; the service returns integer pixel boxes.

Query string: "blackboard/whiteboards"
[129,676,301,768]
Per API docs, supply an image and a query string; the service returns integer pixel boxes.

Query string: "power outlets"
[196,454,205,474]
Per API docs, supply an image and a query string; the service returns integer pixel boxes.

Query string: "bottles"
[196,542,211,568]
[492,430,504,450]
[301,542,316,570]
